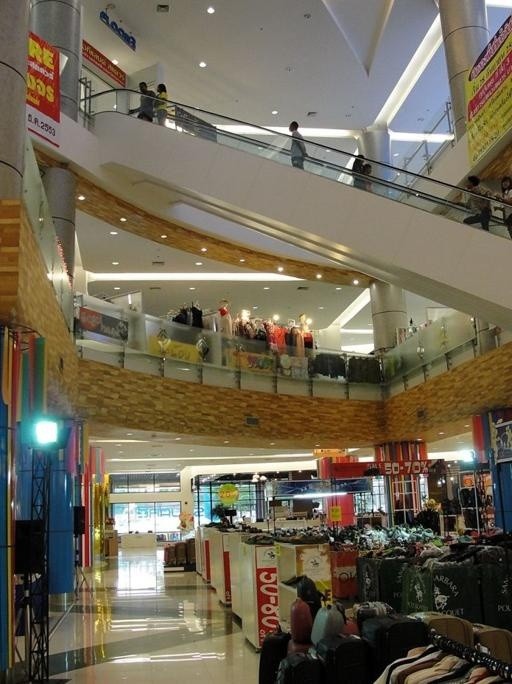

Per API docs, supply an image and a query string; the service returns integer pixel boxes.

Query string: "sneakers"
[204,521,329,546]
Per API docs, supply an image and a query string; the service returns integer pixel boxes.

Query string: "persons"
[129,82,157,122]
[153,84,168,126]
[363,164,373,192]
[289,121,308,170]
[455,175,491,232]
[352,155,366,191]
[494,176,512,240]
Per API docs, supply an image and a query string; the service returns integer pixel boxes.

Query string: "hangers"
[428,629,511,682]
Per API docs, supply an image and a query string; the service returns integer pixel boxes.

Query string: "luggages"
[164,537,196,565]
[258,601,429,683]
[408,610,512,666]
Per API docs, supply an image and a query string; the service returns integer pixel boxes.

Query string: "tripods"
[74,535,93,596]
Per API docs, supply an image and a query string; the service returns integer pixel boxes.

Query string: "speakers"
[74,506,85,534]
[16,520,45,575]
